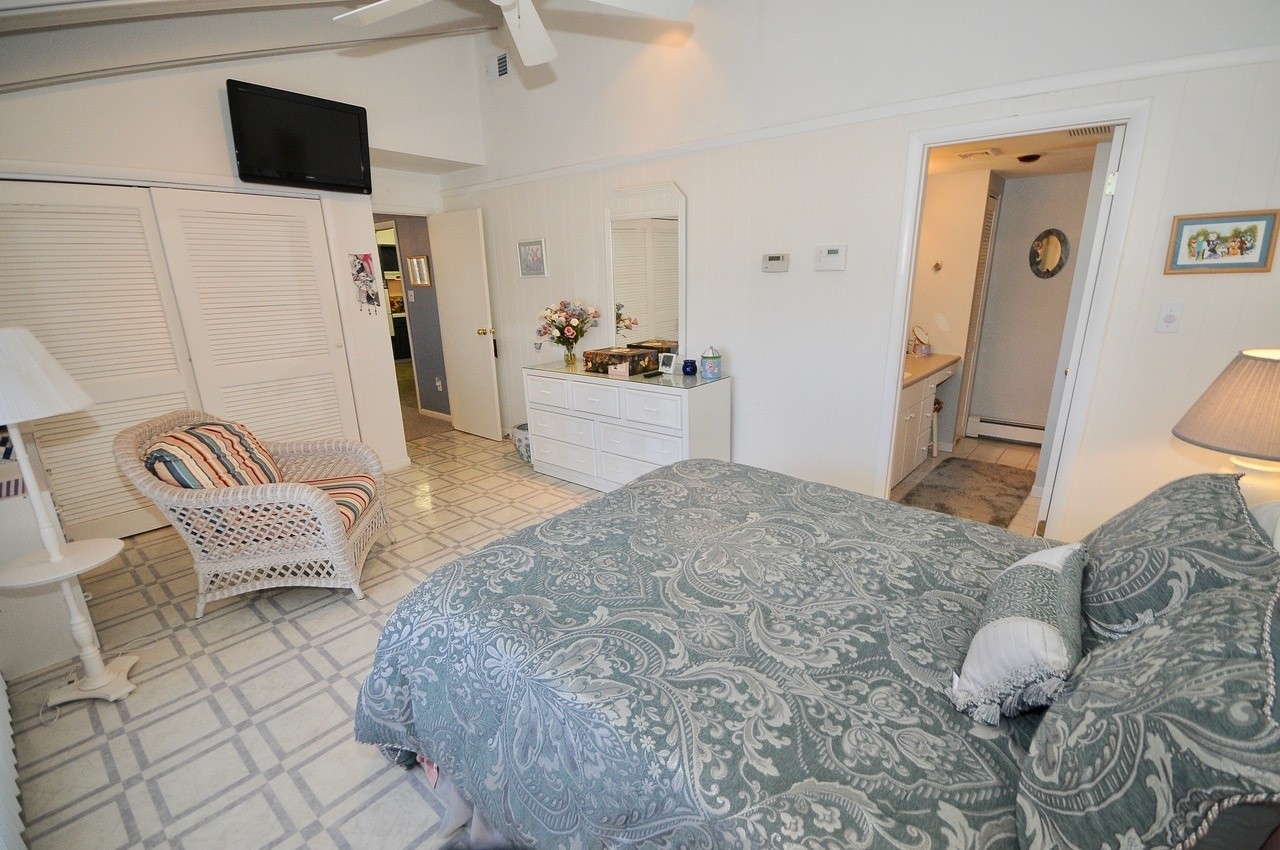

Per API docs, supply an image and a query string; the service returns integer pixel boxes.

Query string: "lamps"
[1171,347,1280,516]
[0,327,139,706]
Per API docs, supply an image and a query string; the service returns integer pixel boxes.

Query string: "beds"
[353,457,1280,850]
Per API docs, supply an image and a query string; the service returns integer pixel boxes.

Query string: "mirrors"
[1028,228,1069,278]
[913,325,931,346]
[605,183,688,358]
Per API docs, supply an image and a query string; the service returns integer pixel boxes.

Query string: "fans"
[332,0,692,67]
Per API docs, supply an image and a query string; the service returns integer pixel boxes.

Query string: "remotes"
[643,372,664,378]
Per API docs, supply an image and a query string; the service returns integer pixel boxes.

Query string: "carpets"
[907,456,1037,529]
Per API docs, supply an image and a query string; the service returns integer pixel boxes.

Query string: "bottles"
[682,360,697,376]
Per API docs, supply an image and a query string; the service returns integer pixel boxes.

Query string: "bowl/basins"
[915,344,931,355]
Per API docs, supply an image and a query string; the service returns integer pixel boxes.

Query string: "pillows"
[1015,575,1280,850]
[955,542,1092,716]
[1077,473,1280,664]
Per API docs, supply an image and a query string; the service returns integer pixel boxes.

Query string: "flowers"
[615,302,638,338]
[535,299,602,347]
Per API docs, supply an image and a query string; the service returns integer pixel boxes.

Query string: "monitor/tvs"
[227,79,371,195]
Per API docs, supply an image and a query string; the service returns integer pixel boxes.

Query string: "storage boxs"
[628,339,678,354]
[583,346,657,375]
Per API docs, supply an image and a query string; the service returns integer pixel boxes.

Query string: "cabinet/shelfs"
[523,362,733,496]
[891,353,960,492]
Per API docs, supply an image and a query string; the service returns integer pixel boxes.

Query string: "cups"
[701,356,722,379]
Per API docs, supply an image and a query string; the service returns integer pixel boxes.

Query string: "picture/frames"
[517,239,548,277]
[1164,210,1279,273]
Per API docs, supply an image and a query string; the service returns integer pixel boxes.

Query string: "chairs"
[113,409,398,615]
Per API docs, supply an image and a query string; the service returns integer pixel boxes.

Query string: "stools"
[927,400,942,457]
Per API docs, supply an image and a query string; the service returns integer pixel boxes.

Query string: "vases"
[564,346,577,364]
